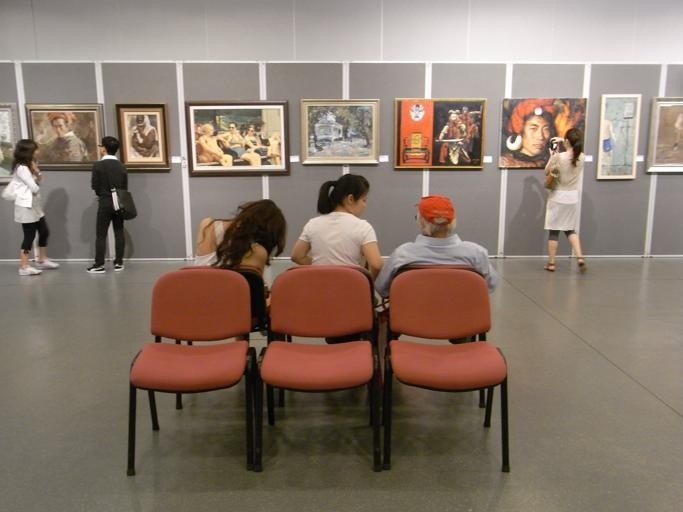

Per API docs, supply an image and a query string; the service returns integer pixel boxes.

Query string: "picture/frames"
[115,103,171,172]
[25,103,105,172]
[394,97,487,169]
[596,93,642,179]
[498,98,588,169]
[301,98,380,166]
[646,96,683,174]
[0,103,23,186]
[185,99,291,177]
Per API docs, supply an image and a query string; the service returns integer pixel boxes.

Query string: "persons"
[10,139,59,276]
[194,199,270,336]
[195,119,282,166]
[374,195,497,376]
[498,99,563,167]
[44,112,89,162]
[437,106,481,165]
[290,174,384,345]
[85,136,128,274]
[543,128,587,273]
[129,115,160,158]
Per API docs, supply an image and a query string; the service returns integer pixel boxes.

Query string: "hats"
[417,195,454,224]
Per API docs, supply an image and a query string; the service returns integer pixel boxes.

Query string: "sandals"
[544,257,584,271]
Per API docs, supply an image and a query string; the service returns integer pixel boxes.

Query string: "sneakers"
[19,259,59,276]
[86,261,123,273]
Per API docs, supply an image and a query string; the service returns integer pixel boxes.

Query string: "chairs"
[380,269,511,473]
[276,263,374,406]
[253,268,382,472]
[175,266,270,410]
[127,268,256,476]
[381,263,486,408]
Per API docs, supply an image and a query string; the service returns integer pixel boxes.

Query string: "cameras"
[546,139,559,150]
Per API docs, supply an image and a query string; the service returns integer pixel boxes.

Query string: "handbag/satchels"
[544,169,558,188]
[112,189,136,219]
[1,180,16,201]
